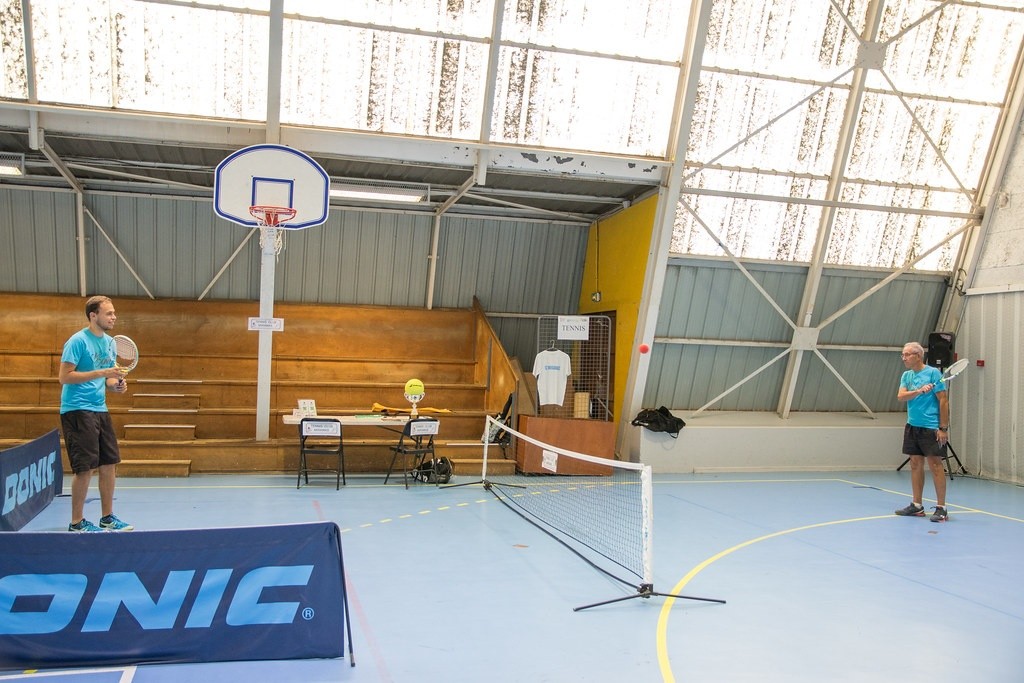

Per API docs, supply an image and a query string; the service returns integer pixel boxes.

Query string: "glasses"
[901,352,918,359]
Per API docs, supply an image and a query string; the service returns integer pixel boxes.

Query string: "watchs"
[939,427,948,432]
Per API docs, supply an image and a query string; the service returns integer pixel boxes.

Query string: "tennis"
[404,378,424,395]
[640,345,648,354]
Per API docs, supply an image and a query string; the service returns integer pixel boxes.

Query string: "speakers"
[927,332,955,368]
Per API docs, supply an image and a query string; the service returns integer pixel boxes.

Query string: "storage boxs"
[516,413,615,476]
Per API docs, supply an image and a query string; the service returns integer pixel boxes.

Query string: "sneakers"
[100,512,134,530]
[68,519,107,532]
[930,506,947,522]
[895,502,925,517]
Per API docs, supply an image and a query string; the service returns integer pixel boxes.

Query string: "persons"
[895,342,949,522]
[59,296,134,534]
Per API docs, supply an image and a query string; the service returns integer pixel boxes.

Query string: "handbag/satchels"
[411,457,452,484]
[632,405,686,438]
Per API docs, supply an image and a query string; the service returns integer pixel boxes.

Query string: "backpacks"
[481,412,512,447]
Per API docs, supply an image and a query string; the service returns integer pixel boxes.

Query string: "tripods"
[897,368,967,480]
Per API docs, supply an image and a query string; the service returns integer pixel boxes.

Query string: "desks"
[282,416,433,467]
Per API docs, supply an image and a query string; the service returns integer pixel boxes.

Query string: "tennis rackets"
[108,334,138,386]
[921,358,970,394]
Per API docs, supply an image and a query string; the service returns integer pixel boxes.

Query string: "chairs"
[384,419,440,489]
[298,418,346,490]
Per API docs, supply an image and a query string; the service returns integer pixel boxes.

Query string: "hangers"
[547,340,559,351]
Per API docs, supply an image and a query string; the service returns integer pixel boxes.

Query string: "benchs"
[0,347,507,470]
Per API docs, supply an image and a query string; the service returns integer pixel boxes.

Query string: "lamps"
[330,176,430,206]
[0,151,26,178]
